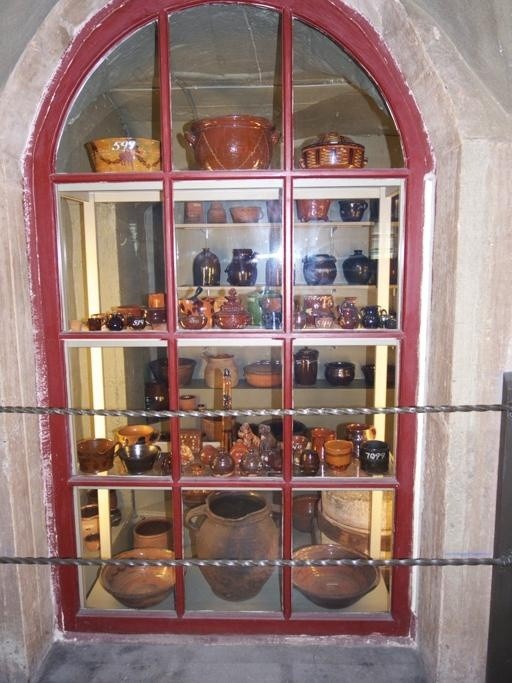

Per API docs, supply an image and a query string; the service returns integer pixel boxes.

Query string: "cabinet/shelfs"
[54,182,406,617]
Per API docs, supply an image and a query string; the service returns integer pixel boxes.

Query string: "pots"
[298,132,367,168]
[184,115,280,171]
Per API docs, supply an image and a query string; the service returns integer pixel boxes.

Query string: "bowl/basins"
[244,360,283,388]
[111,305,144,326]
[325,362,356,386]
[179,315,207,329]
[84,533,100,552]
[361,365,395,388]
[149,358,196,386]
[178,395,197,411]
[116,443,160,473]
[100,547,188,608]
[290,544,381,609]
[129,317,147,329]
[115,424,156,446]
[230,206,263,224]
[84,137,161,173]
[257,419,305,441]
[296,200,331,221]
[77,438,116,472]
[201,417,236,440]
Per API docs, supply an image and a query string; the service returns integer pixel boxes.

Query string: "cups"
[338,199,368,221]
[324,441,354,471]
[94,313,107,327]
[68,319,82,331]
[88,318,101,331]
[184,201,204,223]
[266,200,281,221]
[344,422,376,459]
[359,440,389,474]
[310,426,337,462]
[302,451,319,476]
[269,445,295,472]
[148,292,165,309]
[283,434,309,462]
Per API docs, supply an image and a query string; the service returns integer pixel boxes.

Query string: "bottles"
[293,346,320,386]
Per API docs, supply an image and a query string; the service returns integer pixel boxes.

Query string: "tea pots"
[360,306,381,329]
[315,294,335,328]
[338,296,360,329]
[294,294,307,329]
